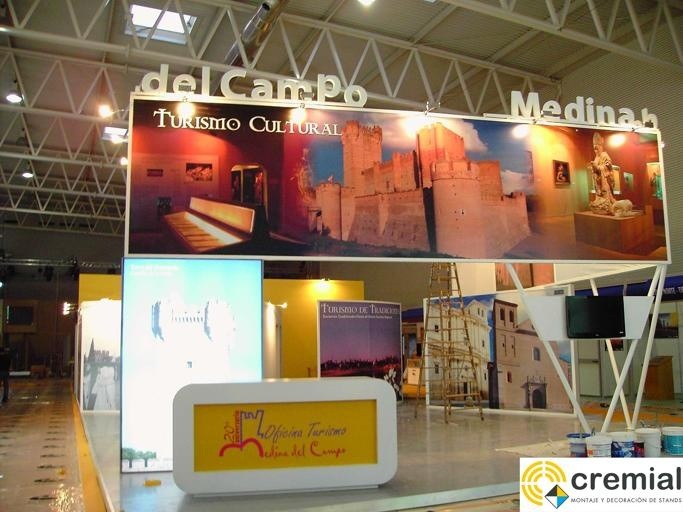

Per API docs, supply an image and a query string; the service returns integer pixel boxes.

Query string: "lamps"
[7,90,22,103]
[22,170,32,178]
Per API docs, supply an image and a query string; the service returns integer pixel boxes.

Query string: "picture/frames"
[172,380,399,498]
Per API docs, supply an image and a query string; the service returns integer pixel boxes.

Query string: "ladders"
[411,264,488,422]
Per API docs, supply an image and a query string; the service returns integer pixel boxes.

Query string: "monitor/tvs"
[564,293,627,338]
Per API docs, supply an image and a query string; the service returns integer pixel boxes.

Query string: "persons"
[585,130,632,212]
